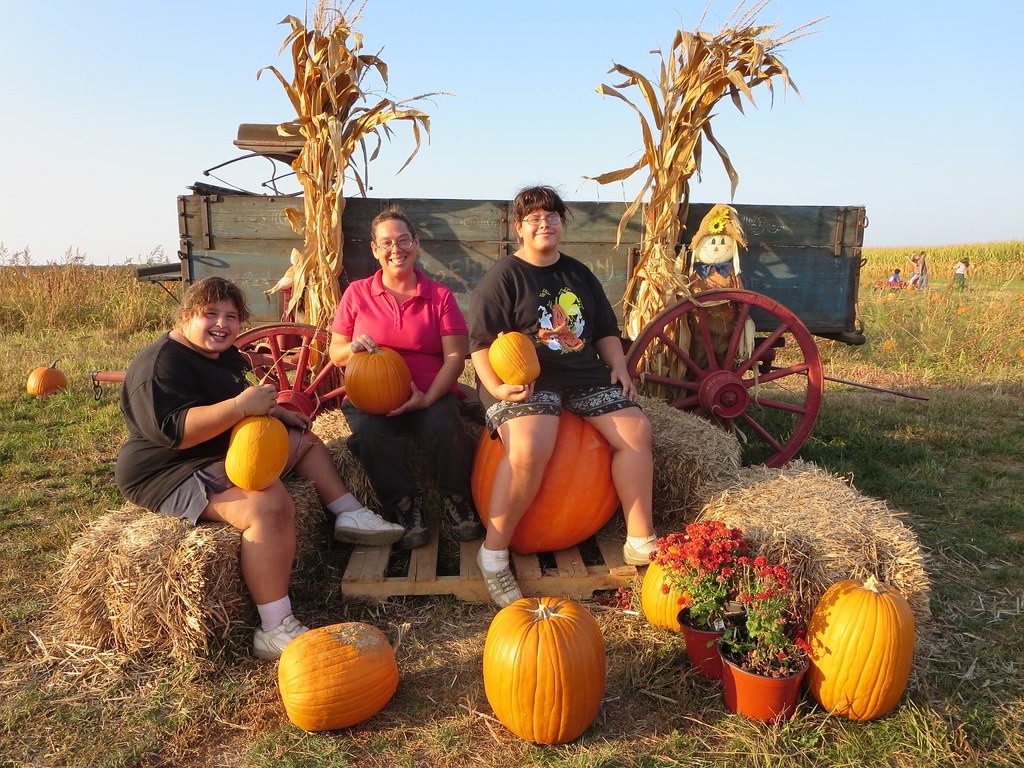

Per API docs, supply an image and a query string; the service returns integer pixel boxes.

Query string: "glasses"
[520,214,562,225]
[371,235,416,252]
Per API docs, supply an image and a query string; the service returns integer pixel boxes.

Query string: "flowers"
[650,517,814,668]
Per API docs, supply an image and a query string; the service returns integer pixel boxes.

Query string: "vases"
[678,605,732,676]
[718,633,811,721]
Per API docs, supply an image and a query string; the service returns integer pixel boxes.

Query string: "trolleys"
[91,182,869,468]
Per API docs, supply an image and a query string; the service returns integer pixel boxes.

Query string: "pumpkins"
[471,405,620,555]
[482,596,605,745]
[803,573,916,721]
[344,347,413,414]
[27,359,68,396]
[278,620,412,731]
[488,330,542,386]
[642,543,701,633]
[225,413,289,490]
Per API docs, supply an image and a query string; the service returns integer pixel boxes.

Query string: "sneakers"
[442,493,482,541]
[393,496,433,550]
[476,545,523,608]
[623,536,662,566]
[334,506,405,547]
[252,613,310,659]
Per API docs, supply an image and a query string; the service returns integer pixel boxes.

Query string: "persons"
[909,252,928,289]
[467,188,659,607]
[115,277,405,658]
[889,269,900,283]
[329,209,482,546]
[955,258,970,278]
[687,205,751,381]
